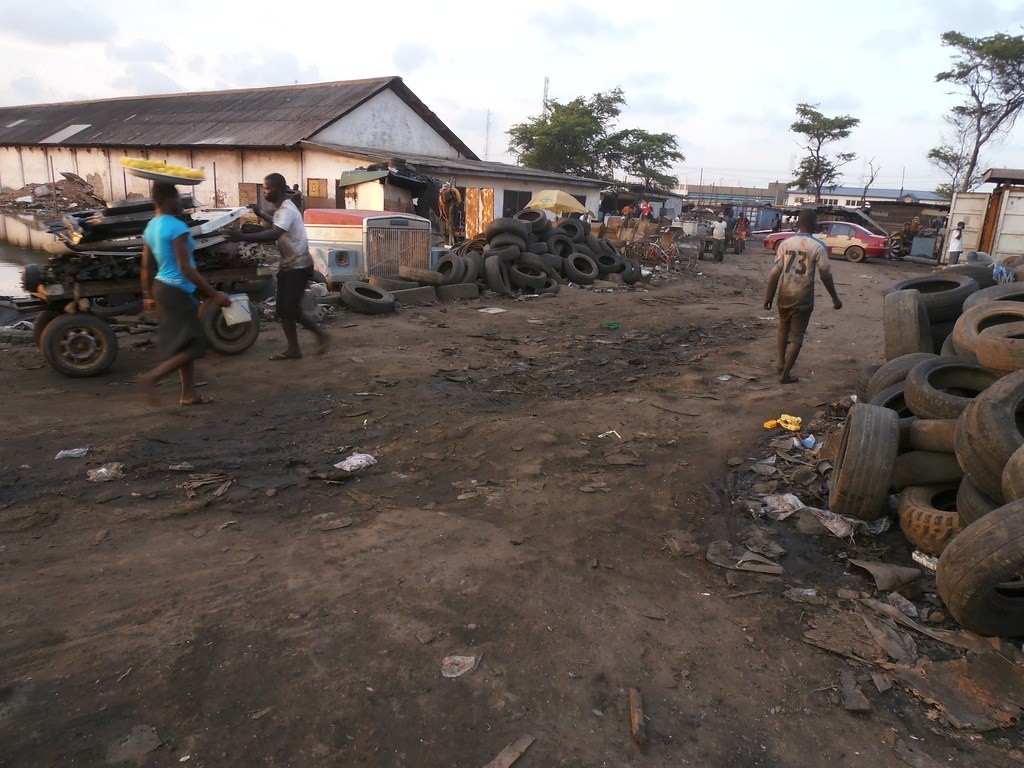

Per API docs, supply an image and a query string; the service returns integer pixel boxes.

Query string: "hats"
[739,212,744,216]
[718,213,724,219]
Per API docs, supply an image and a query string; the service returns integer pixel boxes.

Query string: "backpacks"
[772,218,779,228]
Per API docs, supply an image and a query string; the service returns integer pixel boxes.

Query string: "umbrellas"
[523,189,595,220]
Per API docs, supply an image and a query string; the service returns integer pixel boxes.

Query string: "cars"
[763,221,891,264]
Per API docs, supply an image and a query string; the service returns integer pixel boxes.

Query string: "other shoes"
[774,356,786,371]
[779,373,798,383]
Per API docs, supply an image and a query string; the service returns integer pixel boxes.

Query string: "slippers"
[269,351,290,360]
[314,333,334,354]
[180,396,214,406]
[136,373,153,409]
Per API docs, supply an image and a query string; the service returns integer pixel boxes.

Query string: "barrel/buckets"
[221,293,252,327]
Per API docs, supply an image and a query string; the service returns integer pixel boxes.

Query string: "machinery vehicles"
[12,176,273,377]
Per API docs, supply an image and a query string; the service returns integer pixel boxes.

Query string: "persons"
[221,173,333,359]
[136,182,231,406]
[579,212,591,223]
[948,221,966,264]
[293,184,303,199]
[605,204,655,220]
[724,210,750,249]
[709,212,728,264]
[762,209,842,384]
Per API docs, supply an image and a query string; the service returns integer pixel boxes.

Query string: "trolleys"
[727,232,744,255]
[698,236,724,262]
